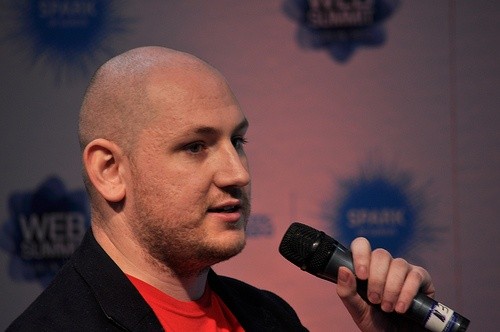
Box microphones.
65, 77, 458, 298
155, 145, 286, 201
278, 221, 470, 331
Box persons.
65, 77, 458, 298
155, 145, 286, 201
0, 45, 439, 331
